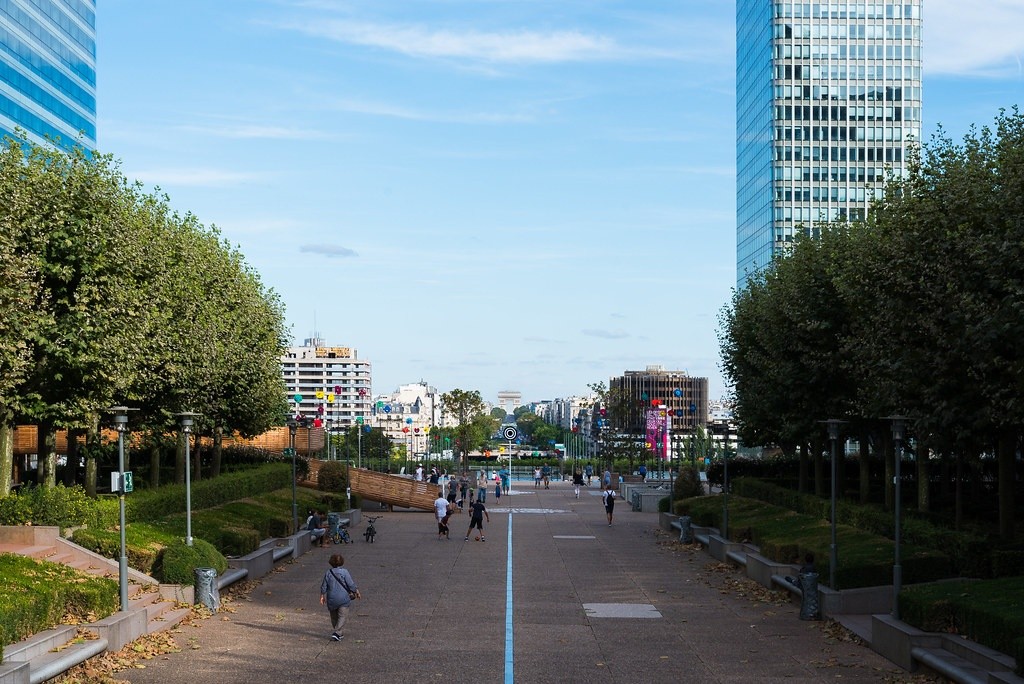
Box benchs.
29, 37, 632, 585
310, 534, 318, 542
339, 516, 350, 526
694, 534, 709, 546
771, 573, 803, 604
218, 567, 248, 589
28, 636, 109, 684
272, 546, 294, 565
726, 550, 748, 567
911, 646, 1011, 684
671, 521, 681, 530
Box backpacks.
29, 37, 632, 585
639, 466, 646, 473
605, 490, 615, 508
604, 471, 610, 483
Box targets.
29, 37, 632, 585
503, 426, 517, 440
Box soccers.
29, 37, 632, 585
475, 535, 480, 541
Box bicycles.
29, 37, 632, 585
362, 514, 383, 543
333, 524, 353, 544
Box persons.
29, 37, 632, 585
320, 554, 361, 641
468, 488, 474, 507
604, 468, 610, 489
477, 469, 488, 503
637, 464, 647, 481
434, 492, 451, 540
299, 414, 322, 428
586, 462, 592, 485
541, 463, 550, 489
306, 509, 330, 548
603, 485, 616, 527
464, 499, 489, 542
494, 481, 501, 505
413, 463, 448, 485
447, 475, 459, 511
573, 470, 584, 499
492, 471, 498, 480
534, 465, 541, 487
499, 464, 510, 496
459, 472, 472, 502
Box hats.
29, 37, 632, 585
469, 488, 474, 491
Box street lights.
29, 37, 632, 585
175, 410, 206, 546
340, 425, 355, 510
106, 407, 141, 612
716, 424, 737, 540
878, 414, 917, 623
818, 418, 852, 588
285, 417, 305, 535
669, 427, 678, 514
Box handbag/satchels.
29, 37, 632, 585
349, 590, 356, 600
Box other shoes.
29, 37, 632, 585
609, 525, 611, 526
482, 538, 485, 542
332, 632, 340, 641
464, 537, 468, 542
322, 543, 329, 547
341, 634, 343, 639
